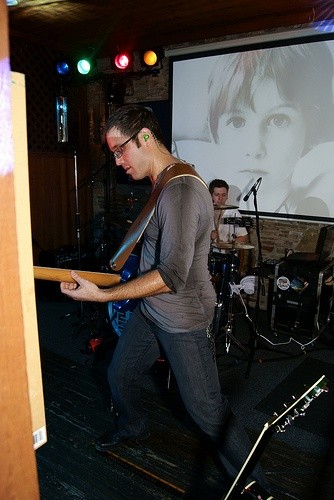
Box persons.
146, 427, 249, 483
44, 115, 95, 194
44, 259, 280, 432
59, 105, 285, 500
209, 180, 248, 245
208, 41, 334, 214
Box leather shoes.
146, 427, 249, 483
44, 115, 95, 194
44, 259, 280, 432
96, 424, 130, 453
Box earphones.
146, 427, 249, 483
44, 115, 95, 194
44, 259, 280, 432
144, 135, 148, 142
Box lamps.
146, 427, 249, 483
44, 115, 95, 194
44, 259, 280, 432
56, 47, 162, 76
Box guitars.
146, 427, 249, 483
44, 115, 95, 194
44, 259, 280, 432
33, 247, 139, 313
220, 373, 333, 500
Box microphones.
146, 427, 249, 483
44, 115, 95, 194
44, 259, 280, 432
243, 178, 262, 202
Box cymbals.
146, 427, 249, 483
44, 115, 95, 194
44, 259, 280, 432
210, 240, 255, 252
213, 200, 239, 211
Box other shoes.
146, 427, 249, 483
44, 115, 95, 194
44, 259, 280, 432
237, 318, 249, 345
216, 342, 230, 354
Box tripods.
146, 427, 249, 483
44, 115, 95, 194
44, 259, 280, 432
215, 190, 292, 380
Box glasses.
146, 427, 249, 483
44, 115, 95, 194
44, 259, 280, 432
114, 131, 139, 158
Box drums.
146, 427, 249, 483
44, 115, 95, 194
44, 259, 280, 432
208, 252, 241, 277
